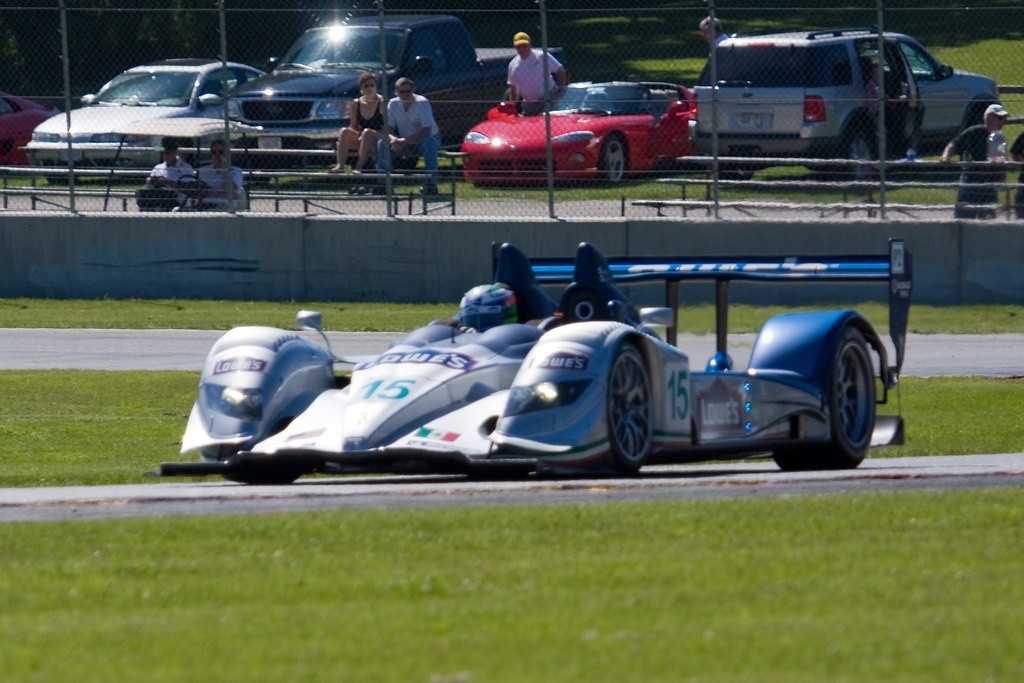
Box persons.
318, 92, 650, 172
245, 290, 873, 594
699, 16, 728, 44
375, 77, 441, 195
507, 32, 566, 116
459, 284, 517, 334
140, 136, 194, 211
329, 72, 383, 174
196, 139, 243, 212
940, 104, 1009, 220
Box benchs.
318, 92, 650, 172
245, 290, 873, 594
0, 147, 468, 216
631, 152, 1024, 219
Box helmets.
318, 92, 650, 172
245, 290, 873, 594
459, 283, 517, 333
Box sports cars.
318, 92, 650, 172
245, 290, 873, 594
461, 78, 697, 185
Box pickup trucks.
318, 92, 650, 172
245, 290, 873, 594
228, 12, 566, 187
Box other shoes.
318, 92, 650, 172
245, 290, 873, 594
327, 165, 345, 174
351, 169, 362, 174
371, 186, 393, 195
421, 183, 439, 199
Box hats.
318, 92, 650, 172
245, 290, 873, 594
983, 103, 1011, 120
513, 31, 530, 46
154, 135, 183, 152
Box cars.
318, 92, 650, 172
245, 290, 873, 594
24, 58, 271, 190
0, 94, 66, 167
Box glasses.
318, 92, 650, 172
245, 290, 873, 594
211, 149, 226, 156
516, 44, 528, 50
362, 83, 374, 87
399, 89, 411, 95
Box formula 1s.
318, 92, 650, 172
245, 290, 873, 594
150, 240, 922, 483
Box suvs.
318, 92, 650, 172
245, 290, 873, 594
686, 27, 1005, 190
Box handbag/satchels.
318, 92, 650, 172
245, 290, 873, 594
136, 176, 179, 209
178, 173, 214, 199
